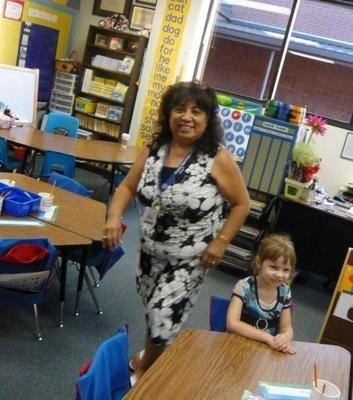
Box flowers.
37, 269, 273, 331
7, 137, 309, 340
288, 115, 328, 186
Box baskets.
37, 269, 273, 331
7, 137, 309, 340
0, 186, 42, 217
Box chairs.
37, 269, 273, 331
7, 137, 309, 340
73, 322, 131, 400
209, 296, 295, 332
0, 112, 104, 342
96, 165, 130, 202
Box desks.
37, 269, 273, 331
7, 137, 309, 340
308, 186, 352, 221
122, 328, 352, 400
0, 127, 143, 193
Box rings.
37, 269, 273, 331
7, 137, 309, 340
103, 236, 106, 240
211, 260, 216, 266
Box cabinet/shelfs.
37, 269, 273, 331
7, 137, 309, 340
218, 196, 283, 280
74, 24, 148, 142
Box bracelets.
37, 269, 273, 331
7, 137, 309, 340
218, 234, 229, 244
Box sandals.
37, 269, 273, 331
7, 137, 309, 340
129, 376, 138, 387
128, 349, 145, 371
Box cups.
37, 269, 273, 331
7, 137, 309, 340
121, 133, 130, 148
0, 178, 17, 188
37, 191, 55, 213
308, 379, 341, 400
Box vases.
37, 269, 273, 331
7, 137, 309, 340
285, 177, 314, 201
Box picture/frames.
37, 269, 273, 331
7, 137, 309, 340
92, 0, 133, 20
134, 0, 158, 7
129, 4, 155, 32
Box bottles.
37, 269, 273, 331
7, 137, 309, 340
301, 178, 318, 205
314, 189, 326, 205
265, 99, 306, 124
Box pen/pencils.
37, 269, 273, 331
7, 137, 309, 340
1, 191, 11, 200
314, 361, 317, 388
50, 180, 56, 197
8, 169, 16, 184
322, 384, 326, 393
0, 191, 5, 198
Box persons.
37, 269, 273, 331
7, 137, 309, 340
102, 80, 250, 383
226, 235, 296, 357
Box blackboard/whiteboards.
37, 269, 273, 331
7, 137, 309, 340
0, 63, 40, 127
